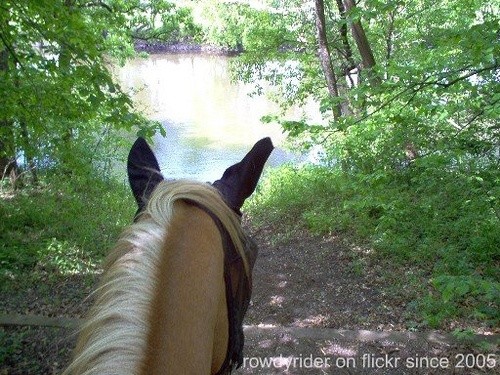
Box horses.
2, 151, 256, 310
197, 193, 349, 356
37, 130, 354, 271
56, 136, 276, 375
